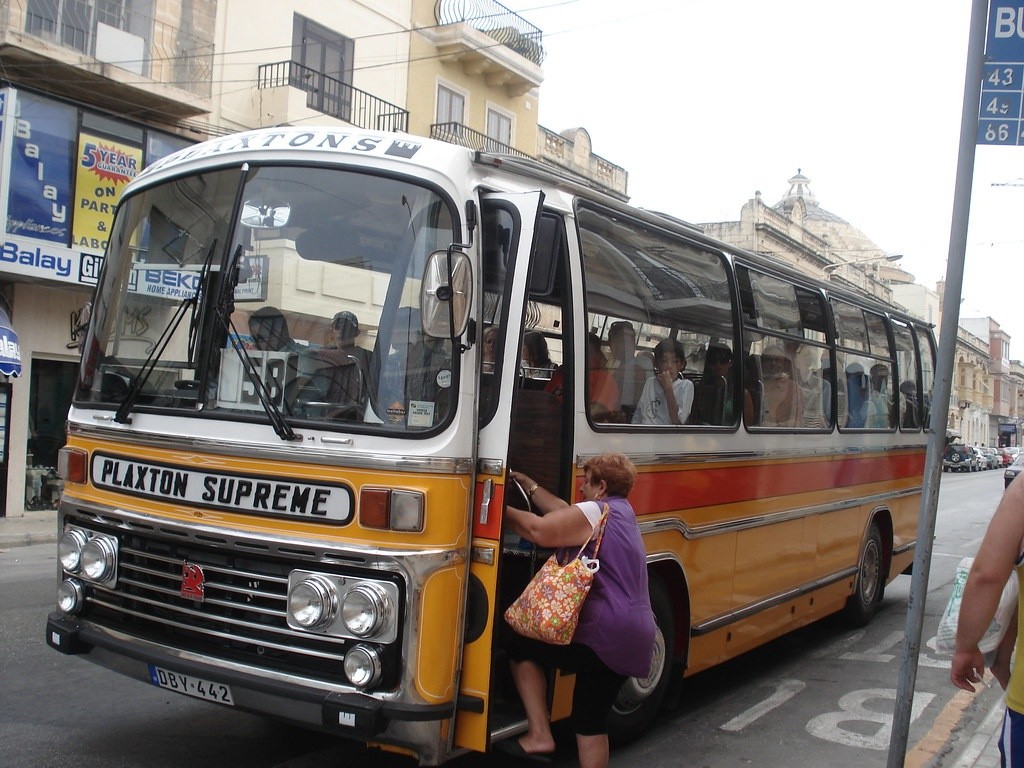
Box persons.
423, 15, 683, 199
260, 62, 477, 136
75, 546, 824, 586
493, 452, 656, 768
248, 306, 329, 416
321, 312, 373, 365
974, 442, 979, 447
950, 467, 1024, 768
483, 321, 931, 429
981, 443, 985, 447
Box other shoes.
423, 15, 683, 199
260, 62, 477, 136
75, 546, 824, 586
495, 740, 551, 761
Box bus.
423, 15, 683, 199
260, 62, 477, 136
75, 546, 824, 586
46, 126, 938, 768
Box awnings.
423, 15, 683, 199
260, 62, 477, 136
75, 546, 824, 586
0, 292, 23, 378
946, 429, 961, 443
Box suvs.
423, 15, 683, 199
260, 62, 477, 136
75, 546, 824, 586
942, 442, 979, 472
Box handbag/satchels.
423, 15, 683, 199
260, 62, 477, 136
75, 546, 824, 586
502, 503, 610, 645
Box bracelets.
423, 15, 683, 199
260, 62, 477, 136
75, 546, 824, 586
527, 483, 538, 499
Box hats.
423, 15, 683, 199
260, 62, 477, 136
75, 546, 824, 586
331, 311, 358, 328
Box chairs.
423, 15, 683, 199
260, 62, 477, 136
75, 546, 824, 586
481, 361, 556, 389
687, 355, 918, 429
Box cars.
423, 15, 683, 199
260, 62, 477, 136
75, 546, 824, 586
973, 448, 987, 471
981, 448, 999, 469
989, 448, 1004, 468
998, 448, 1013, 468
1004, 452, 1024, 489
1005, 447, 1020, 461
